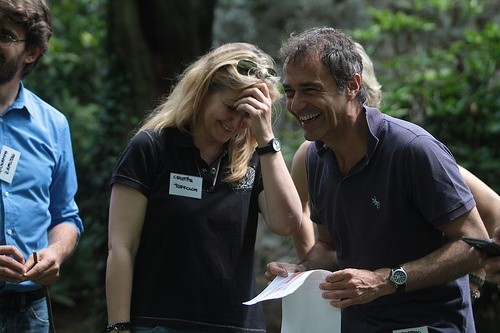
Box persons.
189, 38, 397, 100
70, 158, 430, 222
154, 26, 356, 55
263, 24, 488, 333
0, 0, 84, 333
286, 42, 500, 333
101, 43, 304, 333
469, 224, 500, 291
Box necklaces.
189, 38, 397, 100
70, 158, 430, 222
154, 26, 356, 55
193, 153, 221, 199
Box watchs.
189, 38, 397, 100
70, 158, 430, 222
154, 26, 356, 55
388, 263, 407, 294
255, 137, 282, 155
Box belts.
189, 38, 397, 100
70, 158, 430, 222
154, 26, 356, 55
1, 287, 46, 307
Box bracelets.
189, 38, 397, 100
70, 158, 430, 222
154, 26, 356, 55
106, 322, 132, 332
472, 290, 481, 299
469, 273, 485, 288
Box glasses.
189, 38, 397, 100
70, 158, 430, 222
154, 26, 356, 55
0, 33, 25, 47
233, 59, 278, 80
